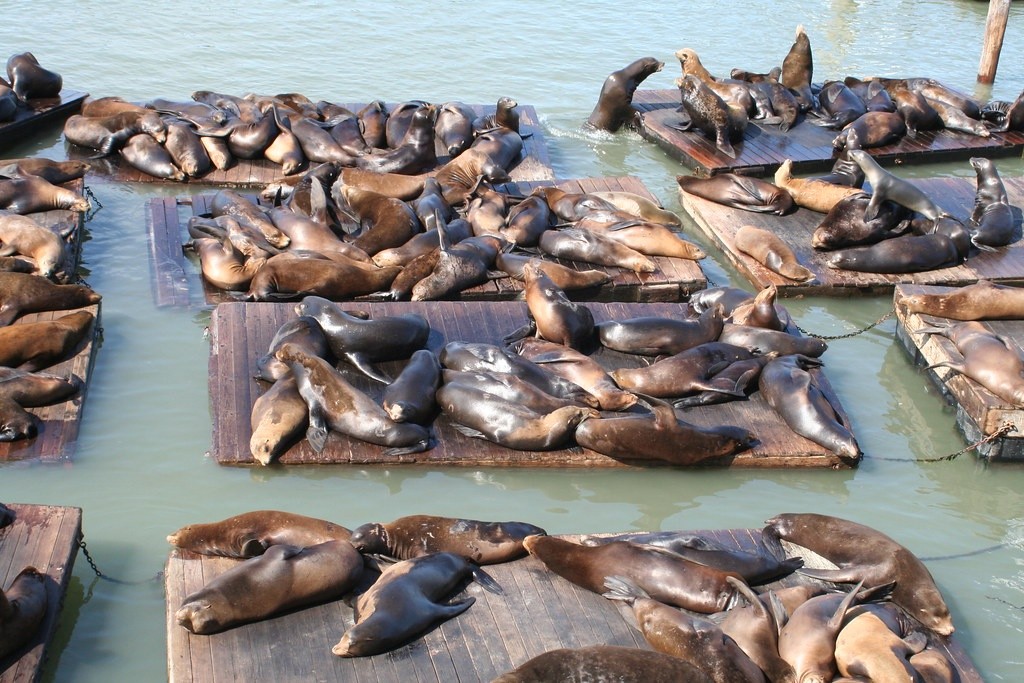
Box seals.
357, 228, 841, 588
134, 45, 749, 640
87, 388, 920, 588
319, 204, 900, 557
0, 31, 1023, 683
